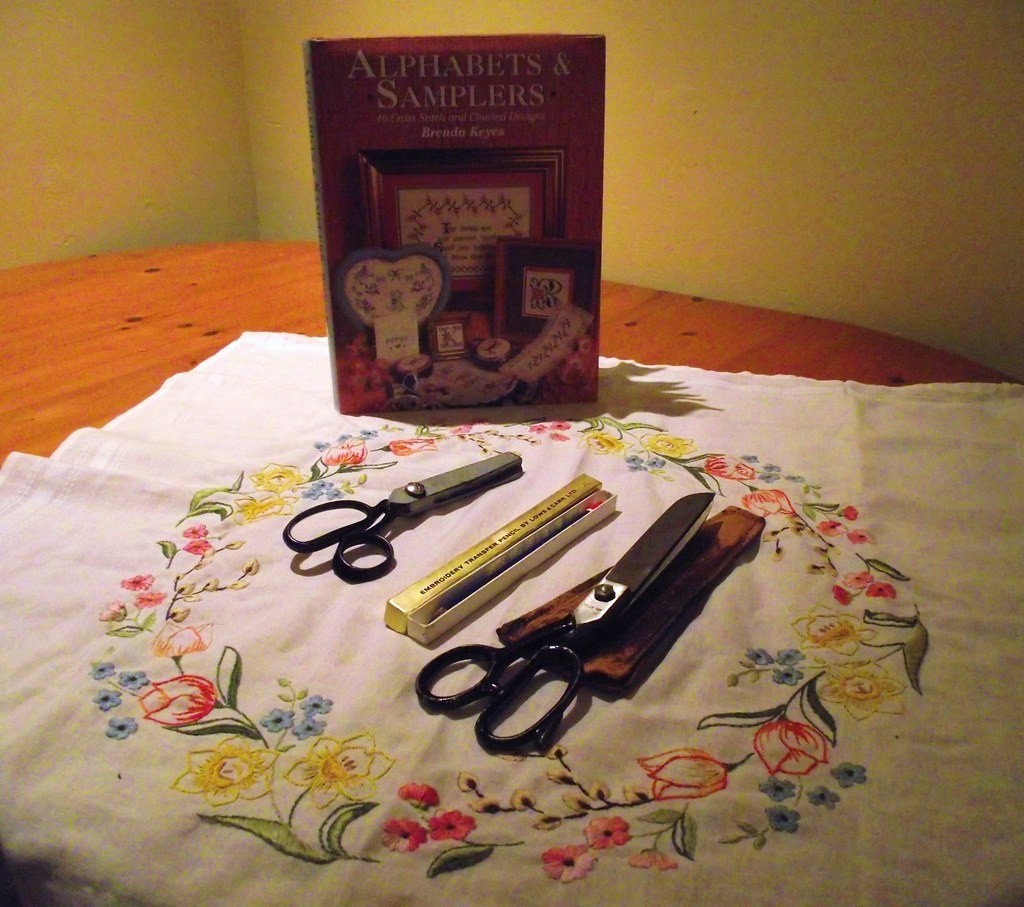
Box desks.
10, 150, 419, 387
0, 240, 1024, 907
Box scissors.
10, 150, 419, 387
281, 450, 523, 584
411, 490, 717, 756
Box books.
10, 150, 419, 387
304, 32, 606, 416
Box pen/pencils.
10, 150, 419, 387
425, 493, 608, 624
